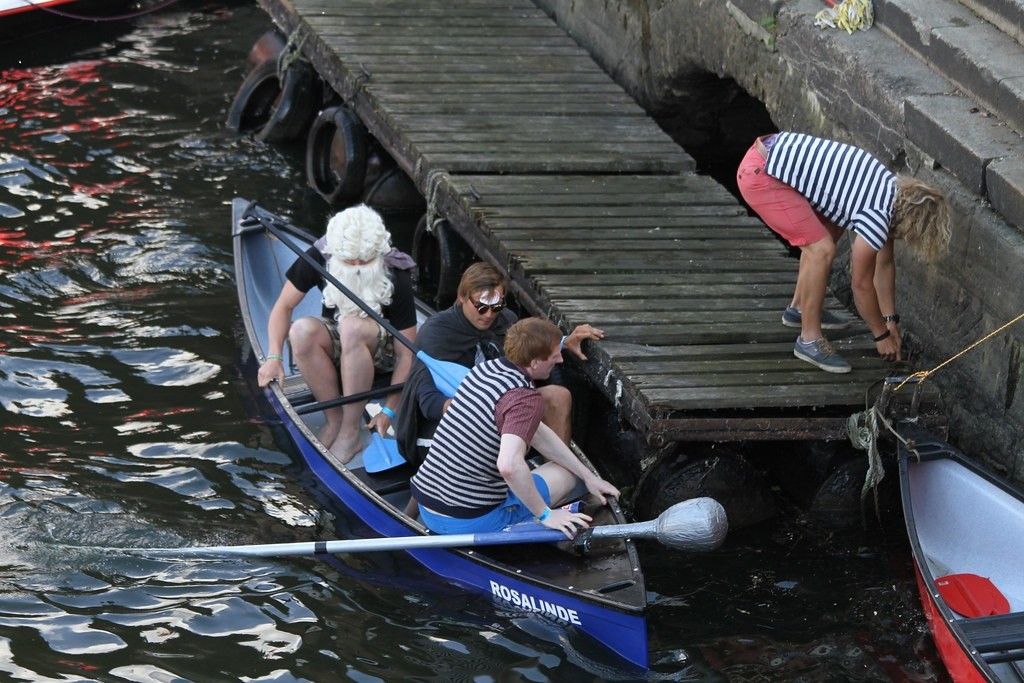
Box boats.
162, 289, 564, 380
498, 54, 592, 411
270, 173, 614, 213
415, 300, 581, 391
229, 197, 650, 671
879, 376, 1024, 683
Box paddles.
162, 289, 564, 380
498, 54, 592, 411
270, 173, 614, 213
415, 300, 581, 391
360, 407, 407, 474
238, 199, 471, 398
934, 573, 1024, 683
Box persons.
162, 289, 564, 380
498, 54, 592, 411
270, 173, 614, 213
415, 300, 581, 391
736, 130, 953, 374
396, 261, 605, 469
256, 203, 418, 465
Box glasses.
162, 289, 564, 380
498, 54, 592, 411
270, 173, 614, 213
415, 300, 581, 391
468, 295, 507, 314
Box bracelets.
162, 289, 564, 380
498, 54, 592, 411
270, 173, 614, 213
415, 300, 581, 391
267, 354, 283, 359
381, 406, 395, 418
872, 330, 890, 342
883, 314, 901, 323
407, 317, 621, 541
560, 335, 567, 349
537, 508, 552, 524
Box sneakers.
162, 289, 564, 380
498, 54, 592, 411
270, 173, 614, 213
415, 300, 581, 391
793, 336, 851, 373
782, 305, 851, 330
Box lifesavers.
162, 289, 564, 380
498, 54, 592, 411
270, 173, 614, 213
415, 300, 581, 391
225, 54, 319, 147
411, 210, 462, 307
307, 108, 366, 205
